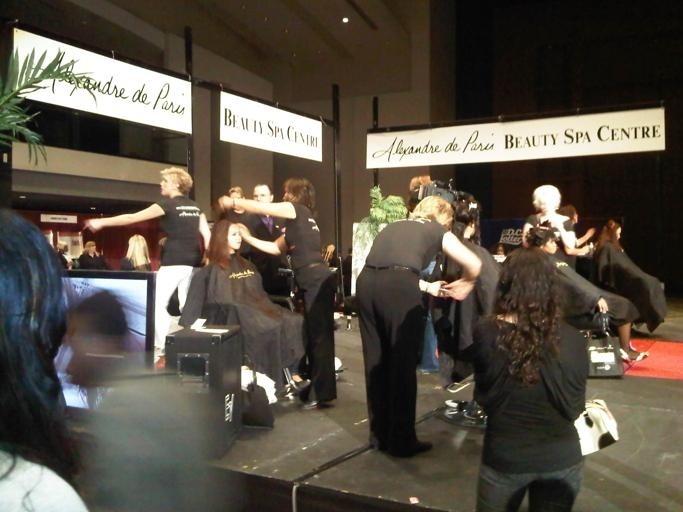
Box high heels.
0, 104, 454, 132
620, 344, 650, 367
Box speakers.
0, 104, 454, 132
165, 324, 242, 458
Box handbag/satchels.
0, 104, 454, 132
584, 336, 625, 378
576, 311, 623, 339
574, 398, 620, 457
239, 353, 277, 429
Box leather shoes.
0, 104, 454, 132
292, 377, 310, 391
302, 399, 321, 411
369, 438, 434, 458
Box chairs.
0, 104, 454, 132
152, 262, 312, 431
563, 308, 626, 379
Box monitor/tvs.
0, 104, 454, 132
52, 269, 156, 422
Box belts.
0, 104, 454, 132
293, 262, 321, 272
364, 264, 420, 277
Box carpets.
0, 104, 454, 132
617, 336, 682, 382
